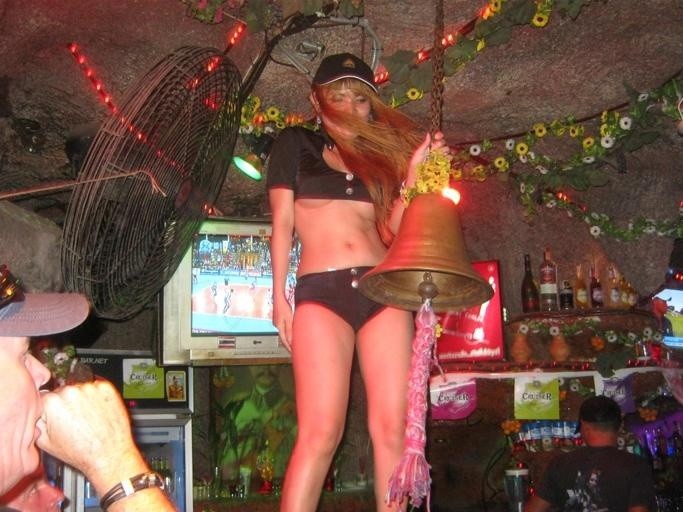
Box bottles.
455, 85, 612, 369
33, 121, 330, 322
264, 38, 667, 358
521, 254, 539, 313
574, 265, 589, 310
590, 264, 604, 308
147, 456, 174, 497
538, 247, 560, 312
559, 280, 574, 311
503, 468, 530, 512
608, 276, 640, 311
209, 466, 224, 499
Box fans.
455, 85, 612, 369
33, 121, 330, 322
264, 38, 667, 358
0, 45, 246, 321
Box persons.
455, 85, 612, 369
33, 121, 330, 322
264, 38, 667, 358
0, 265, 178, 512
268, 53, 453, 512
6, 473, 66, 512
523, 395, 654, 512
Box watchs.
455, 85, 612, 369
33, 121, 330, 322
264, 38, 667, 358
99, 470, 174, 512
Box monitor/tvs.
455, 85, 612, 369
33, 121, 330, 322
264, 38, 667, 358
156, 219, 304, 367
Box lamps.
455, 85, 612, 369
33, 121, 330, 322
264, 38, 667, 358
233, 132, 276, 182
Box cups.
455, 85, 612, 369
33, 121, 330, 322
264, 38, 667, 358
239, 465, 252, 497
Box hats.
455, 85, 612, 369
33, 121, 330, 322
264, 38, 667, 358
314, 52, 379, 94
0, 265, 90, 337
574, 395, 621, 434
